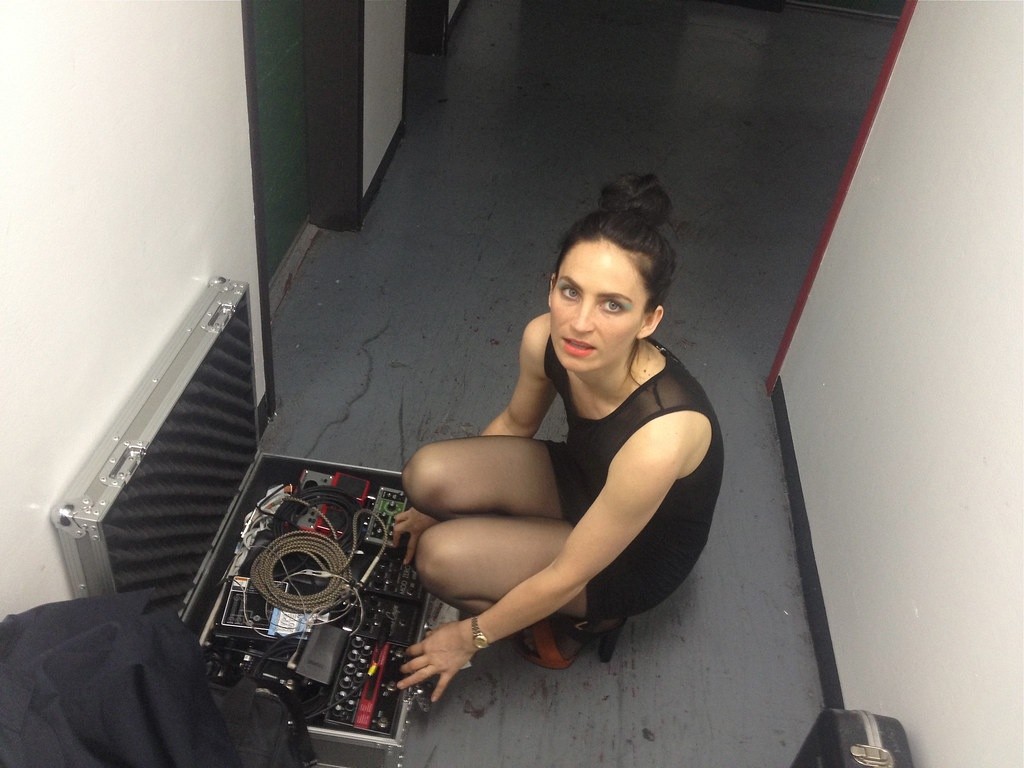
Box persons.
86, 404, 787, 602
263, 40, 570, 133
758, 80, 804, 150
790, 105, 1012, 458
396, 171, 726, 703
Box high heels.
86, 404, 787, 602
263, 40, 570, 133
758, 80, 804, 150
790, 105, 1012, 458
512, 616, 627, 670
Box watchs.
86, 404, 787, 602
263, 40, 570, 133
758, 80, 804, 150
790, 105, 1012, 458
472, 614, 492, 649
